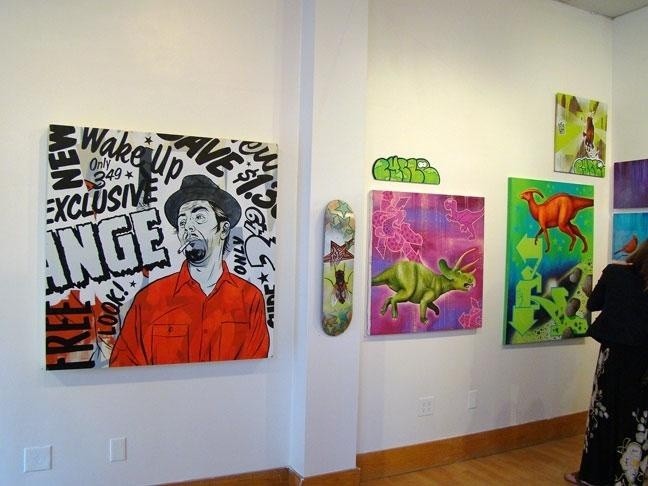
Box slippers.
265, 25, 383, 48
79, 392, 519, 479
564, 470, 588, 486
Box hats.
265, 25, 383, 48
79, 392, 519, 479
164, 175, 242, 231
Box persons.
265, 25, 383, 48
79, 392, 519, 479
565, 237, 648, 485
109, 173, 270, 369
